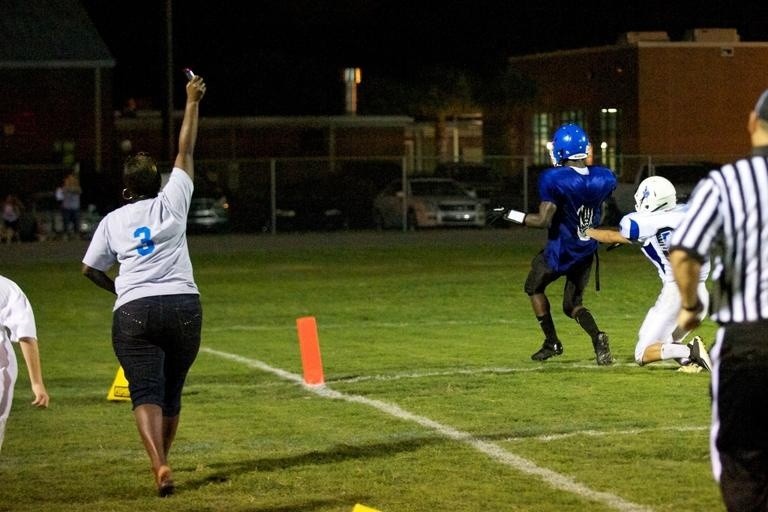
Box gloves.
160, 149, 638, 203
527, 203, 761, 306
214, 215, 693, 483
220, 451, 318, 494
579, 207, 594, 233
486, 207, 510, 224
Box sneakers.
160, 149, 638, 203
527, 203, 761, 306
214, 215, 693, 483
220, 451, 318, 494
532, 342, 563, 360
677, 336, 712, 373
592, 332, 612, 365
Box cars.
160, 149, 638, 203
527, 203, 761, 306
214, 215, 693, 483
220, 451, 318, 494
371, 178, 490, 233
510, 164, 557, 216
233, 177, 318, 234
186, 183, 232, 235
25, 191, 104, 241
302, 173, 377, 230
431, 162, 509, 207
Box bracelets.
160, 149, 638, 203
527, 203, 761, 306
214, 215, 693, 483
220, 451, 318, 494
682, 297, 701, 312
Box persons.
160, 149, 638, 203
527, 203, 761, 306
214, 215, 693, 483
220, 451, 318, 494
578, 176, 713, 373
0, 275, 50, 451
667, 90, 768, 512
56, 174, 82, 242
1, 193, 24, 246
82, 74, 207, 501
493, 124, 618, 366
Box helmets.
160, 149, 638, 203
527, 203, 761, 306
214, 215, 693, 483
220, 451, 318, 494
552, 124, 589, 164
634, 176, 677, 211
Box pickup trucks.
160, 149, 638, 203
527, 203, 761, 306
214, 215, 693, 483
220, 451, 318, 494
605, 160, 709, 227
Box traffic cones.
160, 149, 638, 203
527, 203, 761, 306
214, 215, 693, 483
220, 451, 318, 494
105, 364, 129, 402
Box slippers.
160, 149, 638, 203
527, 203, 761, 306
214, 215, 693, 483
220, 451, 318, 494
159, 480, 174, 497
